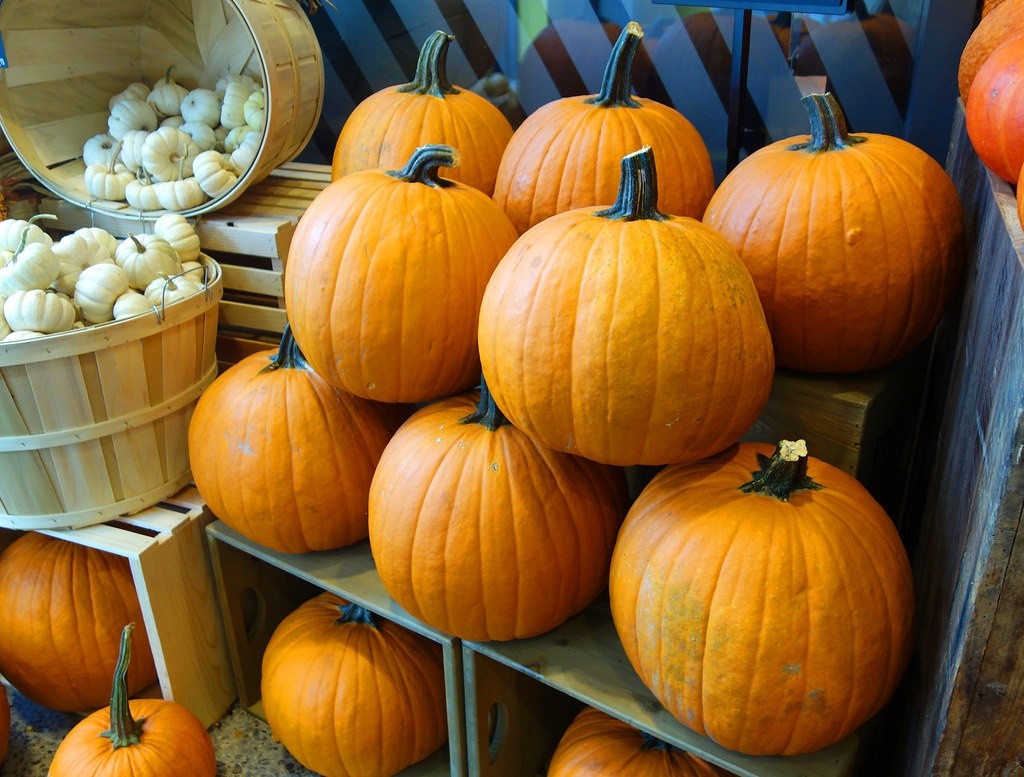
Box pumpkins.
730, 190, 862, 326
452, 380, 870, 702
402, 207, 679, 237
1, 0, 1024, 777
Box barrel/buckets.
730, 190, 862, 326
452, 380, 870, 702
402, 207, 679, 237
0, 251, 222, 530
1, 0, 324, 234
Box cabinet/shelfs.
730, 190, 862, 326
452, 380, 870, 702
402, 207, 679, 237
0, 92, 1024, 775
